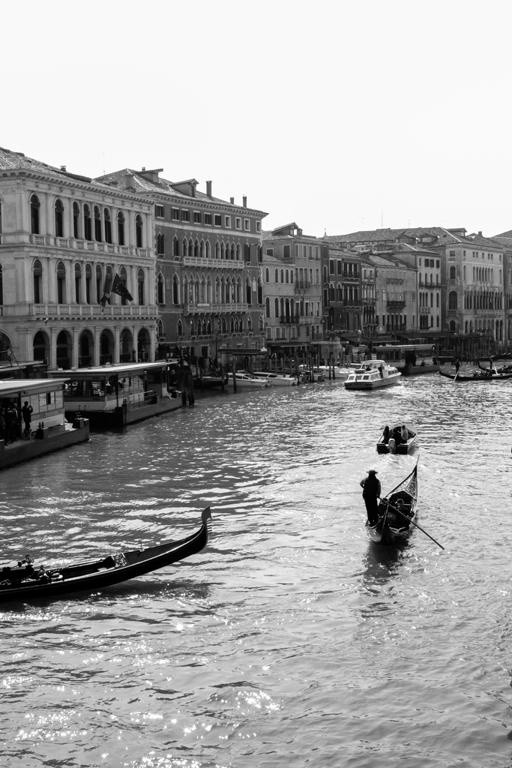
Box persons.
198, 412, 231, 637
382, 425, 409, 445
360, 471, 380, 528
455, 360, 460, 374
366, 365, 370, 370
420, 360, 425, 366
4, 403, 18, 446
22, 401, 33, 438
377, 364, 384, 378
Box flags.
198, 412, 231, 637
111, 273, 132, 301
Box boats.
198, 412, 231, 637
365, 454, 420, 545
0, 505, 213, 599
190, 363, 361, 387
344, 359, 402, 390
376, 425, 417, 453
438, 370, 512, 381
477, 362, 512, 372
